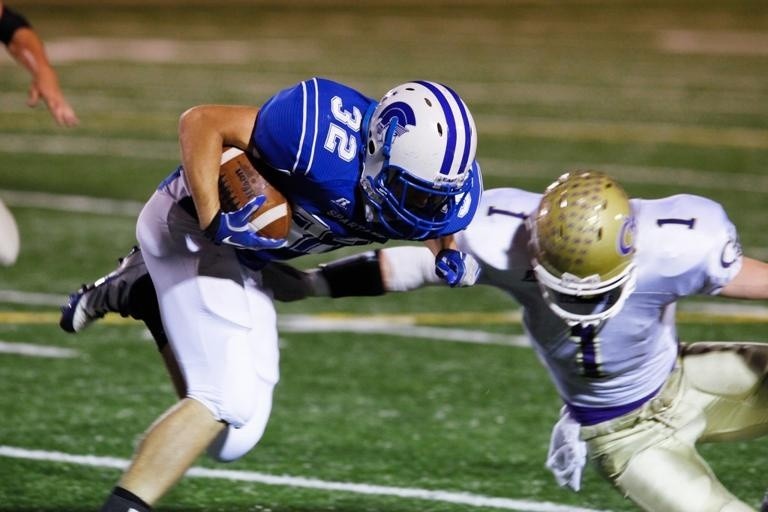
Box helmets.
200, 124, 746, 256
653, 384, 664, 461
356, 77, 485, 218
525, 167, 641, 301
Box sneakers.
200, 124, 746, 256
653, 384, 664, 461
59, 245, 149, 333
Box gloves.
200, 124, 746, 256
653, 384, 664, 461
261, 261, 307, 302
434, 247, 483, 289
200, 194, 289, 252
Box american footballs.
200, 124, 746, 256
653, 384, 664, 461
218, 145, 292, 239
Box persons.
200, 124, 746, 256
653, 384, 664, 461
236, 169, 768, 512
0, 0, 80, 268
59, 77, 483, 512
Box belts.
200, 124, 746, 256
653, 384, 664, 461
577, 352, 685, 443
